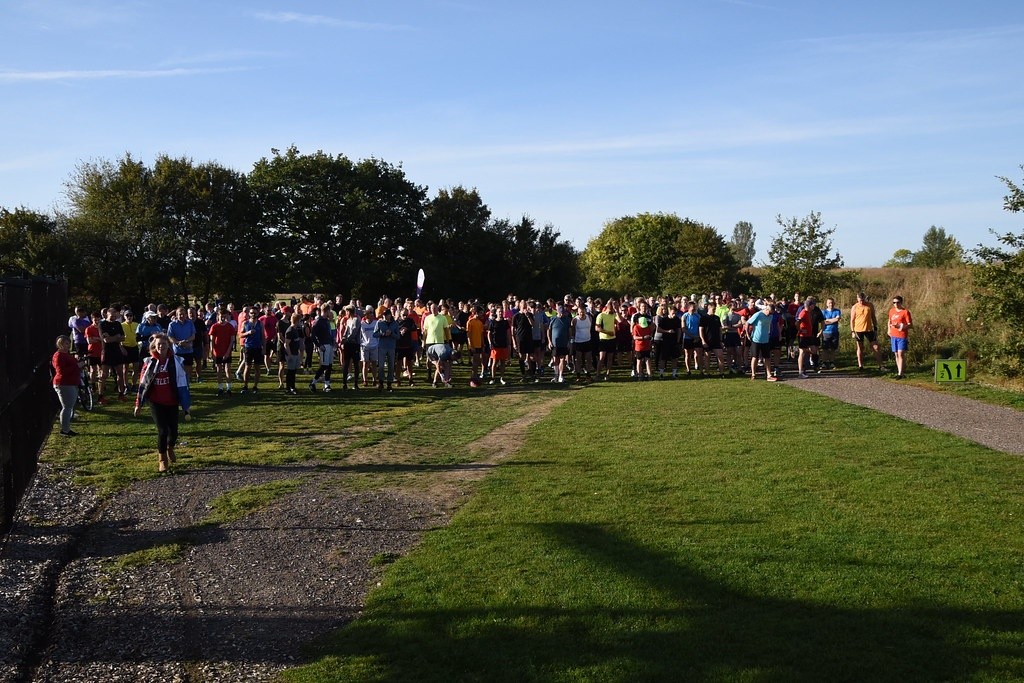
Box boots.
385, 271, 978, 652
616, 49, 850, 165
159, 451, 168, 472
167, 445, 176, 461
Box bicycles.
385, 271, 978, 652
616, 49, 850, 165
69, 351, 94, 412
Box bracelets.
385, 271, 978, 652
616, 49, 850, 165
384, 330, 387, 335
642, 336, 644, 340
898, 323, 903, 331
851, 330, 854, 333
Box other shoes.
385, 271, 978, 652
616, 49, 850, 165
378, 384, 382, 390
218, 389, 223, 396
895, 374, 902, 380
228, 390, 232, 396
60, 430, 78, 437
279, 384, 283, 389
373, 381, 377, 386
387, 386, 392, 390
363, 381, 367, 387
118, 396, 128, 401
98, 398, 109, 405
292, 388, 298, 394
396, 365, 837, 388
343, 385, 348, 391
235, 371, 242, 380
241, 386, 247, 394
858, 366, 865, 373
197, 377, 203, 382
252, 387, 257, 393
309, 383, 316, 393
323, 386, 332, 392
355, 385, 359, 390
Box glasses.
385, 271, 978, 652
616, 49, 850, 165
126, 315, 132, 317
893, 302, 901, 304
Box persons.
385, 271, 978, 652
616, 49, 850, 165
133, 334, 191, 473
53, 290, 914, 435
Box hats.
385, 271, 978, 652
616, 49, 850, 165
145, 311, 158, 318
639, 317, 648, 328
382, 310, 392, 315
124, 310, 133, 316
